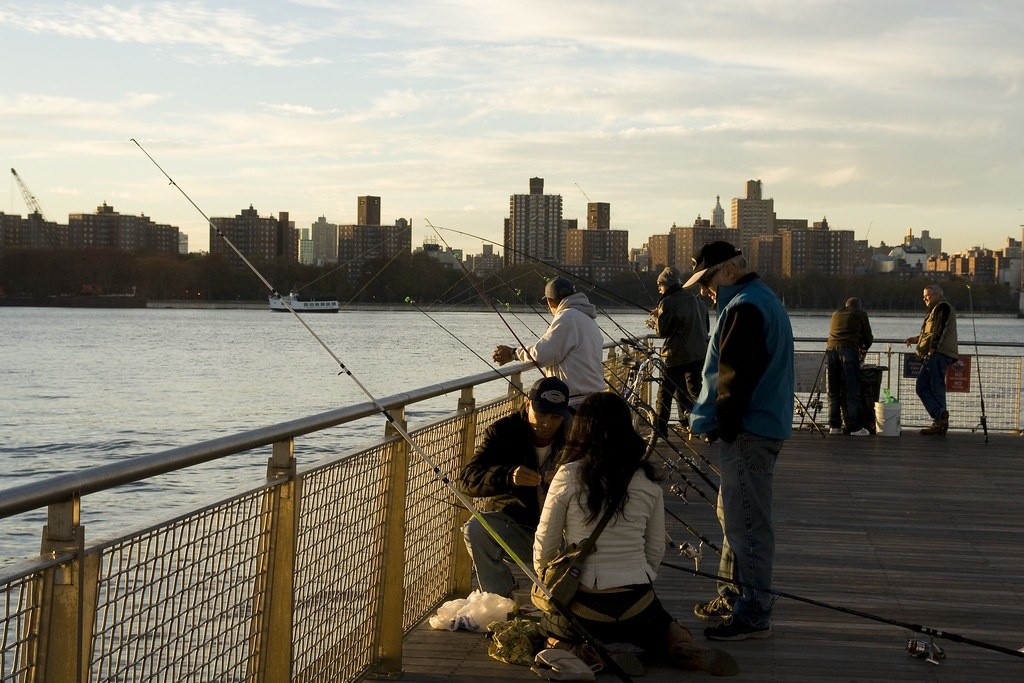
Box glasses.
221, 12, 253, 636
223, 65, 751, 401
700, 264, 725, 288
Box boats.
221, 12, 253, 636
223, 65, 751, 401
268, 292, 339, 313
0, 282, 148, 309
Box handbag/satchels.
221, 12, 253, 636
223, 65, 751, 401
531, 538, 598, 615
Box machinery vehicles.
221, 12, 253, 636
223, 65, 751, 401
10, 168, 82, 296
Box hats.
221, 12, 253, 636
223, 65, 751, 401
541, 276, 573, 299
530, 376, 571, 420
681, 240, 742, 288
657, 267, 680, 286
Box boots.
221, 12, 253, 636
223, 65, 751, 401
553, 641, 647, 676
666, 624, 740, 676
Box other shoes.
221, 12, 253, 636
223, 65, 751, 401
673, 425, 690, 434
920, 420, 942, 434
642, 434, 666, 443
934, 411, 950, 435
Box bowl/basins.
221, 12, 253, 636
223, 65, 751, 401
511, 589, 531, 605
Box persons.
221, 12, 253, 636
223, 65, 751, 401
531, 392, 740, 676
903, 285, 959, 434
825, 297, 873, 436
457, 376, 579, 597
643, 266, 711, 442
680, 240, 796, 640
491, 276, 603, 412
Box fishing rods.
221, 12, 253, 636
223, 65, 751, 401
402, 295, 723, 572
421, 177, 722, 511
791, 218, 875, 438
966, 285, 990, 443
417, 488, 1023, 669
126, 135, 631, 682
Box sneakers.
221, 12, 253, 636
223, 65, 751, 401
829, 428, 843, 435
704, 615, 772, 640
850, 427, 870, 436
694, 595, 735, 620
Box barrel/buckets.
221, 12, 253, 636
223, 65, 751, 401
873, 401, 902, 437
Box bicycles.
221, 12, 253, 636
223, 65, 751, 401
618, 337, 667, 463
